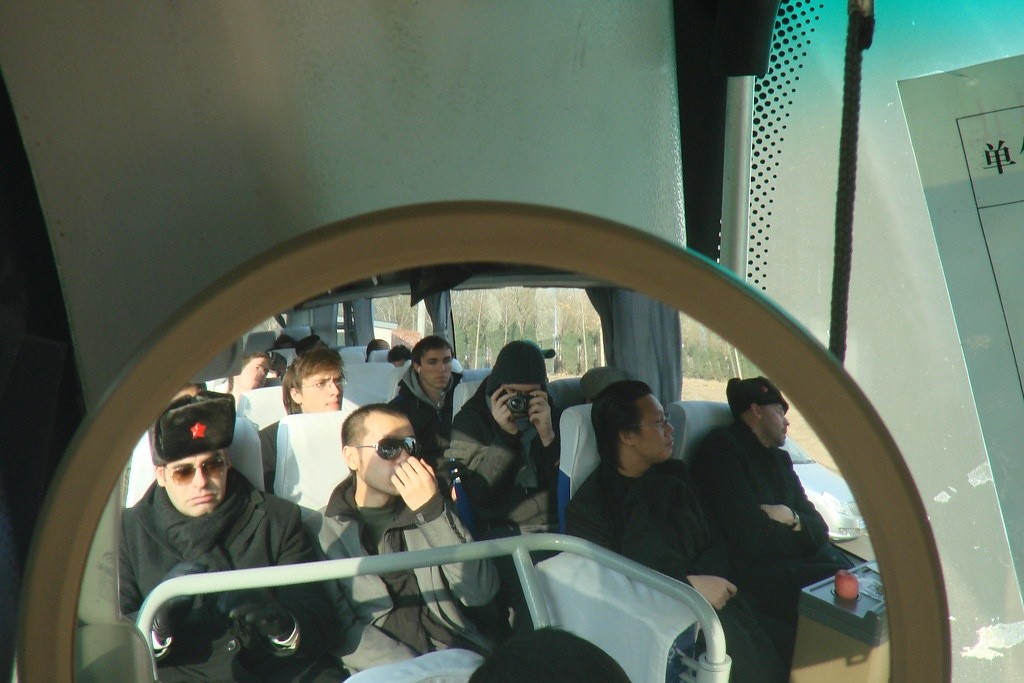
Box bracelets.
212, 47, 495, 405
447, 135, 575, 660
788, 510, 799, 529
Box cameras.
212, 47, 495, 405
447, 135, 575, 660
506, 391, 536, 417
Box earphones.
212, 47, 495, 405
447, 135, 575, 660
417, 367, 421, 375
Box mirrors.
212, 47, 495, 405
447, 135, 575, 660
12, 190, 964, 683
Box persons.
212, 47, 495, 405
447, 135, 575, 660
222, 335, 628, 642
118, 381, 632, 683
565, 375, 830, 683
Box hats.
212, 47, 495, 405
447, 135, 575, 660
148, 390, 236, 466
487, 341, 548, 385
725, 376, 788, 416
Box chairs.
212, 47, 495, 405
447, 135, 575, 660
280, 325, 312, 343
338, 348, 365, 362
338, 361, 399, 406
271, 347, 296, 367
239, 387, 357, 427
245, 332, 276, 356
666, 397, 738, 463
367, 349, 389, 362
548, 376, 589, 405
387, 358, 462, 411
403, 358, 464, 373
460, 368, 503, 384
451, 379, 484, 426
550, 402, 598, 536
124, 416, 266, 508
270, 412, 353, 513
341, 343, 367, 360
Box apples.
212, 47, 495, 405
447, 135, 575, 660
833, 568, 859, 598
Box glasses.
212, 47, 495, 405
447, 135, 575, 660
355, 435, 418, 460
300, 372, 349, 391
632, 413, 671, 429
165, 454, 227, 486
